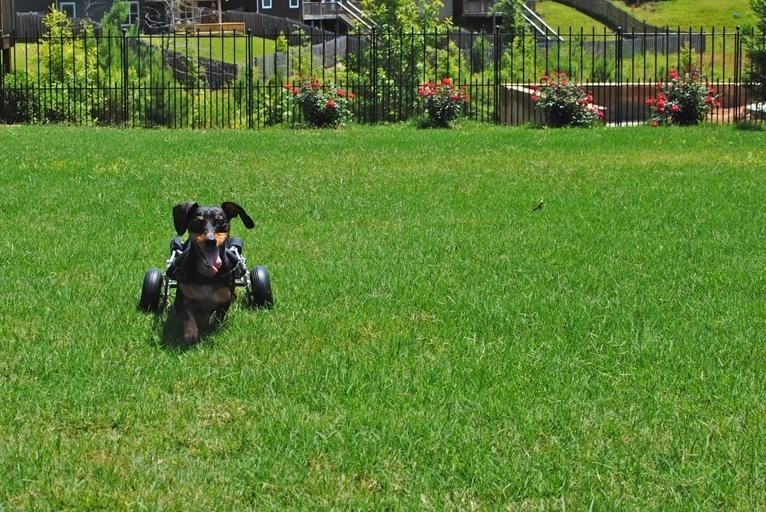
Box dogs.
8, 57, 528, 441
164, 201, 254, 347
